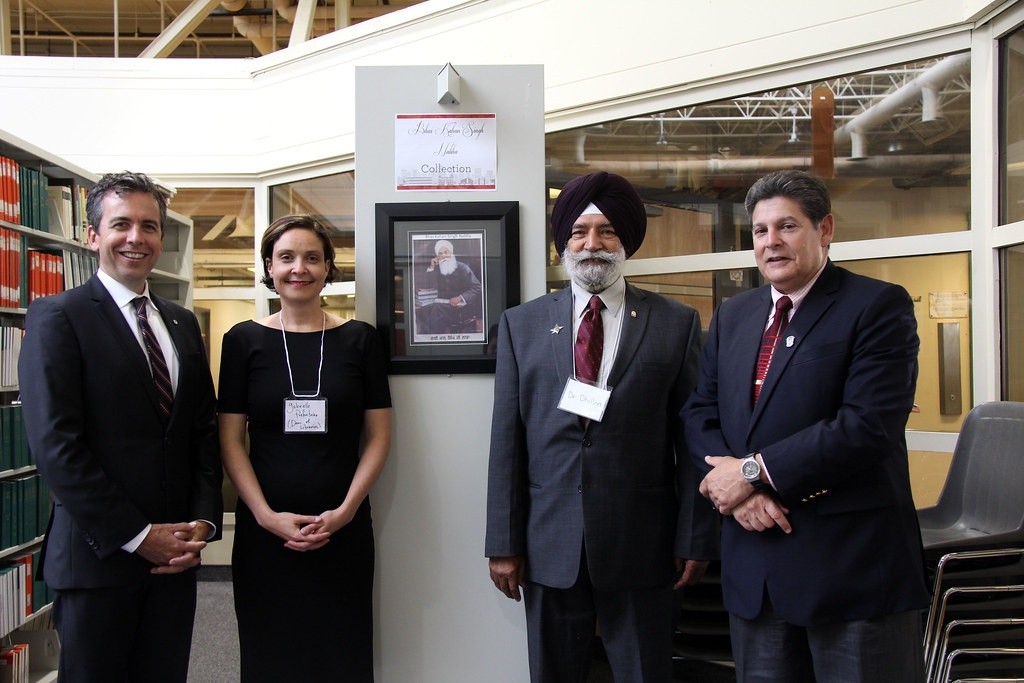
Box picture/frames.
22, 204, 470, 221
375, 201, 520, 374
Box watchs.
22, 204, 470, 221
741, 452, 773, 494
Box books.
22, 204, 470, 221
0, 157, 177, 683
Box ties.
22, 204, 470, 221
751, 296, 794, 411
574, 296, 608, 387
130, 295, 174, 414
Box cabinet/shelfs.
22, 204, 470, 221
0, 130, 191, 683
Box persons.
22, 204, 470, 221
424, 240, 483, 334
485, 172, 703, 683
218, 214, 391, 683
19, 172, 223, 683
683, 169, 932, 683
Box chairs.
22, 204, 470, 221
668, 398, 1024, 683
